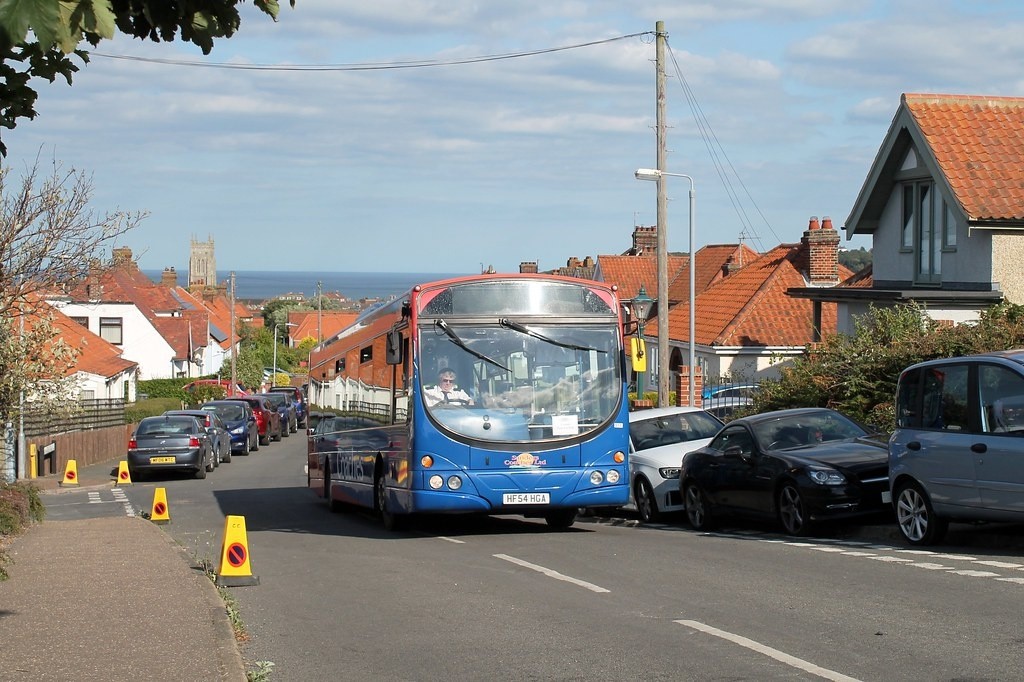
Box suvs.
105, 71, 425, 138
268, 386, 307, 424
160, 410, 232, 467
224, 395, 282, 446
180, 379, 250, 397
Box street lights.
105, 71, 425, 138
272, 323, 298, 387
635, 167, 696, 407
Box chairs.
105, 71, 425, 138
990, 394, 1024, 432
663, 419, 695, 442
147, 426, 165, 435
184, 427, 192, 434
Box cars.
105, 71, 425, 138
701, 383, 760, 409
679, 407, 893, 537
200, 402, 259, 456
254, 393, 298, 437
577, 406, 730, 523
313, 417, 400, 474
128, 415, 215, 481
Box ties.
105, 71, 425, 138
441, 391, 449, 404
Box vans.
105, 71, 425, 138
889, 348, 1024, 547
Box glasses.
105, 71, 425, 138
443, 379, 453, 383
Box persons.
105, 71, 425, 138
404, 368, 474, 406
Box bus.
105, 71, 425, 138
306, 274, 646, 528
